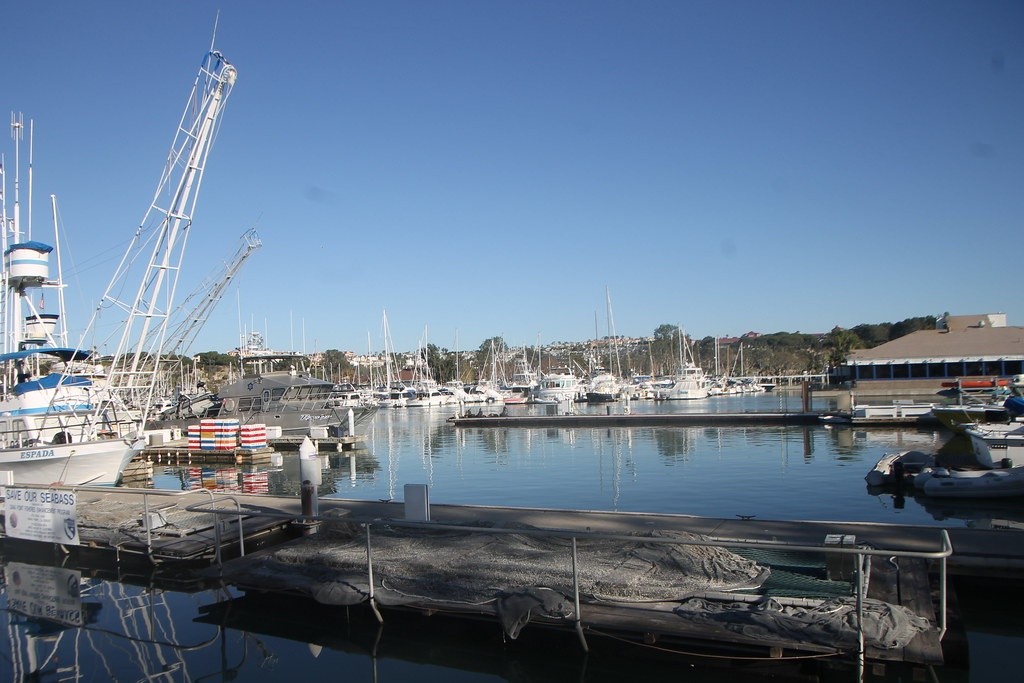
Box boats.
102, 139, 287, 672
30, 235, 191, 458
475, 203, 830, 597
967, 417, 1024, 469
0, 9, 237, 486
930, 375, 1024, 434
138, 311, 379, 438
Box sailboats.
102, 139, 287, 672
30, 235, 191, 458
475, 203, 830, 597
535, 283, 770, 402
332, 309, 534, 408
